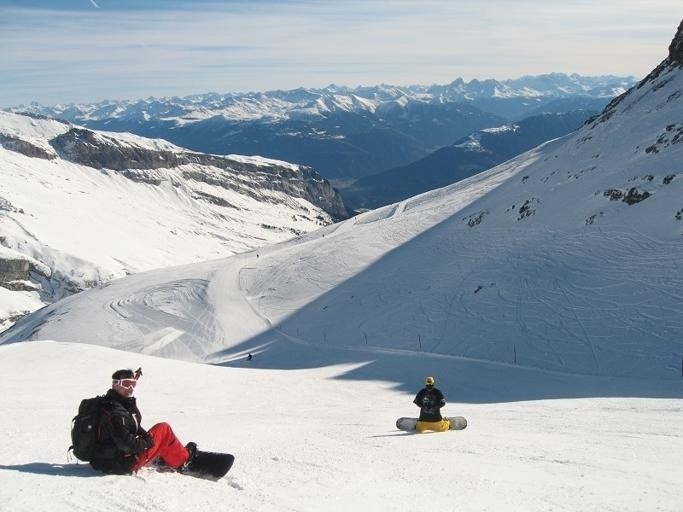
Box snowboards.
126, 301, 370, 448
396, 417, 467, 431
152, 450, 234, 481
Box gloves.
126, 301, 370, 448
143, 435, 152, 448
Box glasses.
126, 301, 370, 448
112, 378, 138, 390
426, 385, 434, 387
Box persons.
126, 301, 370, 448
87, 366, 196, 475
245, 353, 252, 361
412, 374, 451, 434
133, 366, 143, 381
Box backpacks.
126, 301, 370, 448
72, 395, 124, 462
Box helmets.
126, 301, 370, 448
426, 377, 434, 385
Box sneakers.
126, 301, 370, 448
175, 442, 196, 474
